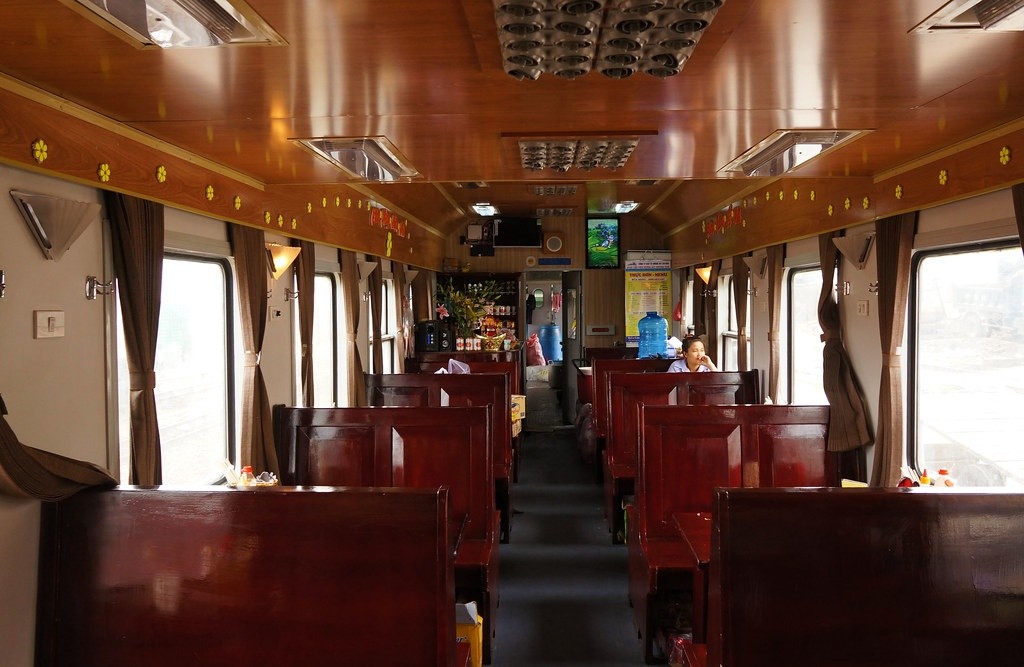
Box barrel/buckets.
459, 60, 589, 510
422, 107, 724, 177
637, 309, 668, 359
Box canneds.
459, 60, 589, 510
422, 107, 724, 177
456, 281, 518, 350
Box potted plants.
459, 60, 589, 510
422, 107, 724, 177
432, 276, 502, 350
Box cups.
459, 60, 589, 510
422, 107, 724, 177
504, 339, 511, 350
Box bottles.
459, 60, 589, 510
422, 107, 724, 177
934, 468, 952, 487
237, 466, 257, 487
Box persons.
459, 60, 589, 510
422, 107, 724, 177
666, 334, 720, 373
526, 287, 537, 340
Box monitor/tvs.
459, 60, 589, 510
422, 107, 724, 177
585, 214, 621, 270
493, 217, 542, 249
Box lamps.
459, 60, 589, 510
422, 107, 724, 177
608, 200, 640, 214
10, 188, 101, 262
264, 242, 302, 297
357, 261, 379, 283
468, 202, 501, 217
694, 265, 712, 285
832, 230, 876, 271
404, 270, 419, 285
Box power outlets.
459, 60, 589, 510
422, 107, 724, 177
33, 310, 66, 339
857, 300, 869, 316
742, 254, 768, 280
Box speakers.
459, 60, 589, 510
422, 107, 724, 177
543, 232, 566, 257
437, 320, 456, 352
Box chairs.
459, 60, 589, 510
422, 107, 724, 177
34, 345, 1023, 667
